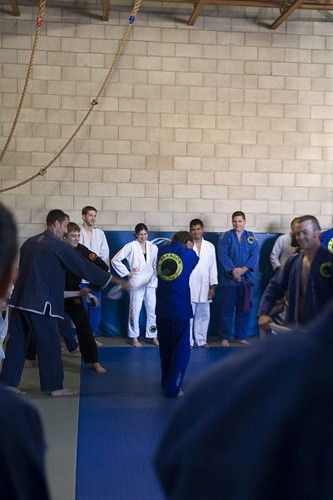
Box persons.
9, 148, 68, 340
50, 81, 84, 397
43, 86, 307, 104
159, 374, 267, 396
0, 206, 333, 500
